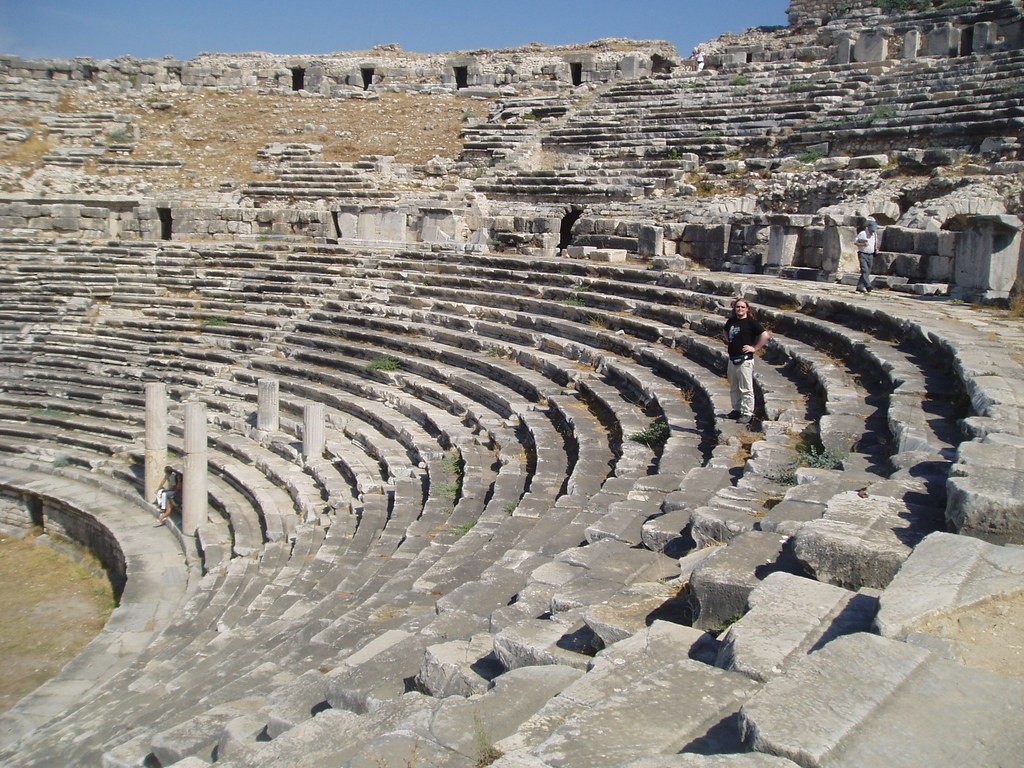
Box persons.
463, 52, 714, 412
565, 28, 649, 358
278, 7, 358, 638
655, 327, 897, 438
854, 223, 877, 292
695, 47, 705, 71
156, 465, 183, 521
722, 298, 769, 423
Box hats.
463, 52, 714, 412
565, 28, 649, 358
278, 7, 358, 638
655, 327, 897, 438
869, 222, 877, 232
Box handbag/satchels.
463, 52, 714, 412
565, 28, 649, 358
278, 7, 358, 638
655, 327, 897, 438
729, 353, 752, 365
874, 249, 878, 257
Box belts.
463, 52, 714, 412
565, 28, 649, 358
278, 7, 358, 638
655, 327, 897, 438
859, 251, 873, 255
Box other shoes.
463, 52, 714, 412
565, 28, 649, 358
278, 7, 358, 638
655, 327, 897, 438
867, 286, 873, 292
737, 414, 752, 423
857, 288, 867, 294
723, 410, 742, 418
